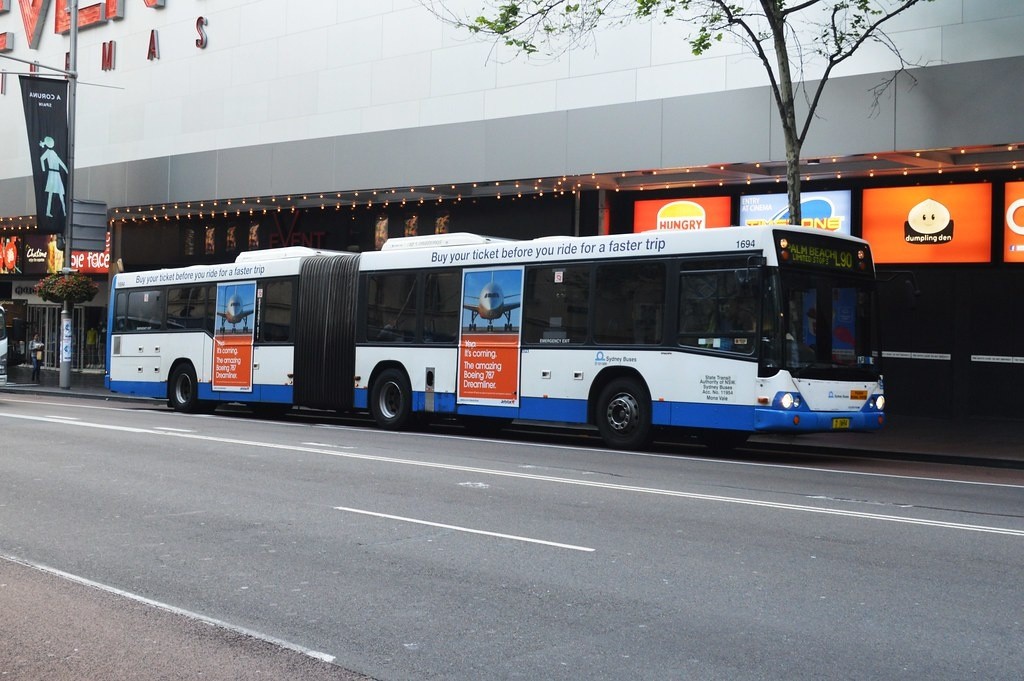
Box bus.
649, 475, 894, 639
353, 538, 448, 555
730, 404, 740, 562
104, 224, 888, 451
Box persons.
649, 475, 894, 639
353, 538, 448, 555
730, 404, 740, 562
29, 333, 44, 383
86, 319, 106, 368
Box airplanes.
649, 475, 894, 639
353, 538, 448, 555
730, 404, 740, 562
464, 272, 521, 332
217, 286, 254, 333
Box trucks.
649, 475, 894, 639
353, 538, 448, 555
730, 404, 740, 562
0, 306, 9, 387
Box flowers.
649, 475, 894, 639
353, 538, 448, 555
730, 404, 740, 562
33, 273, 101, 304
